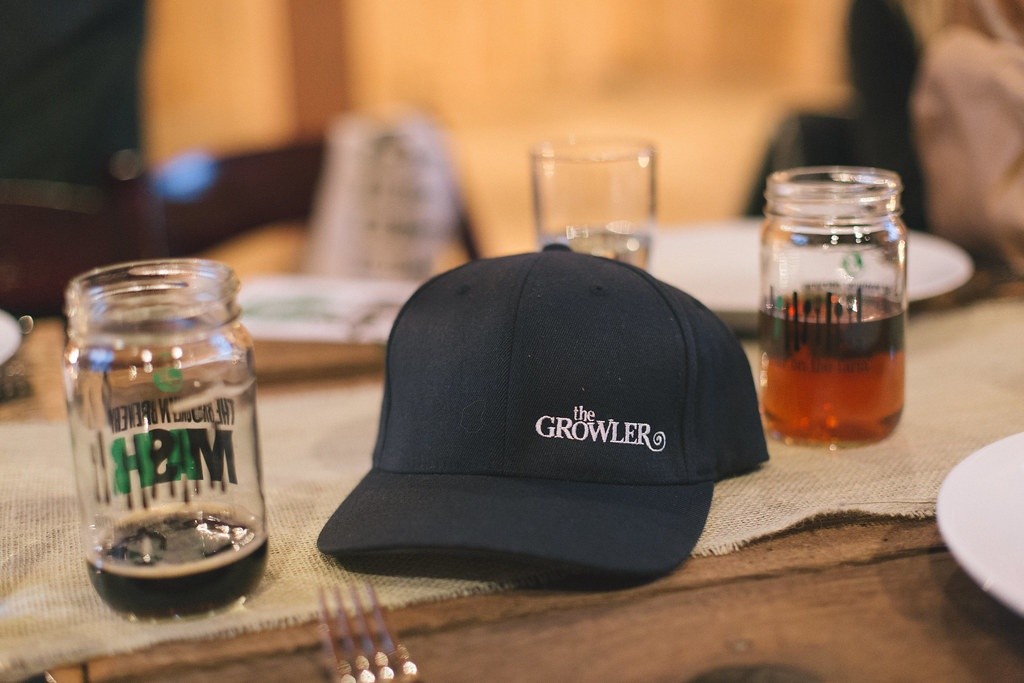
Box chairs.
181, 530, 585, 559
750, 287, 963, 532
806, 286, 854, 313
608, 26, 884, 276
0, 134, 479, 317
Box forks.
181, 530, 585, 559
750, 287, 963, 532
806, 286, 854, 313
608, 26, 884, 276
315, 578, 422, 683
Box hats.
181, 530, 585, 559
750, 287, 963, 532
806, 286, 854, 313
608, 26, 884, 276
316, 243, 770, 572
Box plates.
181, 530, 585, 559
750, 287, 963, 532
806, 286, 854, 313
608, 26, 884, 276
0, 308, 22, 365
937, 432, 1024, 618
655, 221, 976, 339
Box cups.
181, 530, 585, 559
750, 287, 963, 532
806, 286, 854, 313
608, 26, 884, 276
529, 134, 658, 273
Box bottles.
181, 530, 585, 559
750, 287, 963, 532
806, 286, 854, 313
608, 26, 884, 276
758, 164, 910, 450
60, 259, 271, 619
